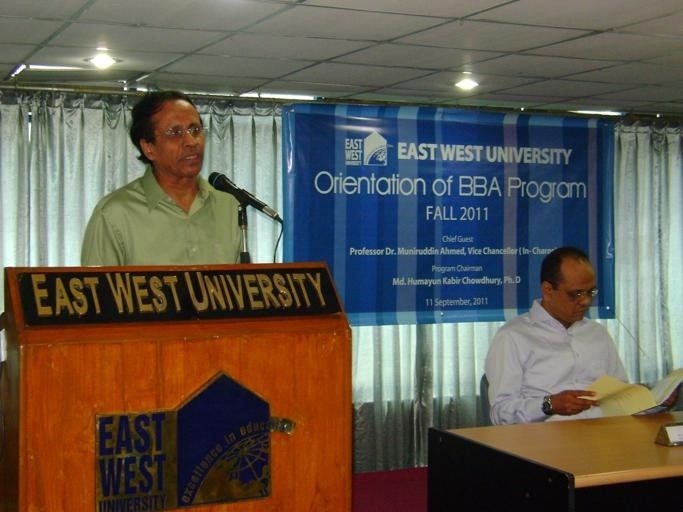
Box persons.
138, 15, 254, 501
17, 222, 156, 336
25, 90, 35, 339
81, 90, 248, 267
484, 246, 679, 426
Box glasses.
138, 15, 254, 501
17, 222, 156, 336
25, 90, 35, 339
556, 285, 598, 300
158, 127, 207, 139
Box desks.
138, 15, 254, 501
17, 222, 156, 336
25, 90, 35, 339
427, 411, 683, 512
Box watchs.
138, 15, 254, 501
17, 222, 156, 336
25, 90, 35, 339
541, 395, 555, 417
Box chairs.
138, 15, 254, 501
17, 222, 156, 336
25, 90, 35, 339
480, 374, 505, 424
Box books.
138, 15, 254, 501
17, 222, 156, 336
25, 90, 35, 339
575, 367, 682, 417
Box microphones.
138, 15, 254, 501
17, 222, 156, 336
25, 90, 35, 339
208, 171, 281, 222
